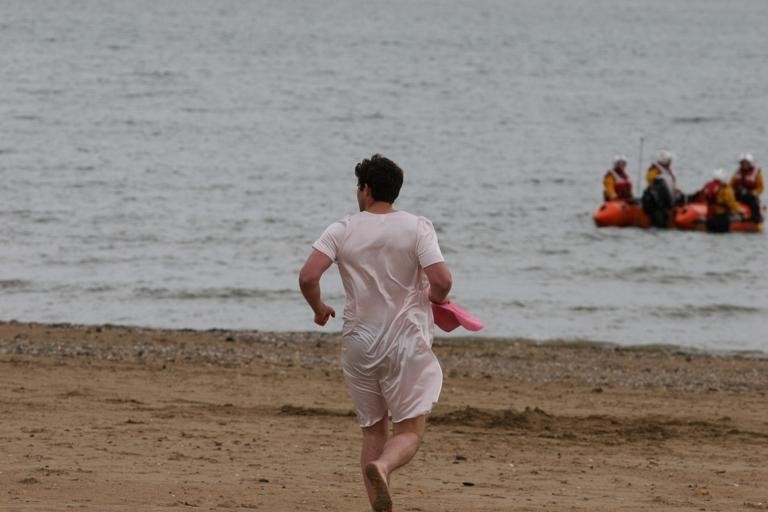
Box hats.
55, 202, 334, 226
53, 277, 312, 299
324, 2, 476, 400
432, 302, 484, 333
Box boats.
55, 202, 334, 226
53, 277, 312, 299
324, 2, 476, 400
593, 197, 765, 232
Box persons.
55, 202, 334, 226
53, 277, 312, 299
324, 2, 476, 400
641, 174, 675, 231
728, 152, 766, 232
601, 154, 639, 206
701, 167, 746, 235
646, 150, 686, 207
298, 152, 454, 512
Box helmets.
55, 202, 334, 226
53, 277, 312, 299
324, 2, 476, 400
614, 156, 627, 169
714, 169, 727, 185
740, 154, 753, 167
657, 151, 672, 164
